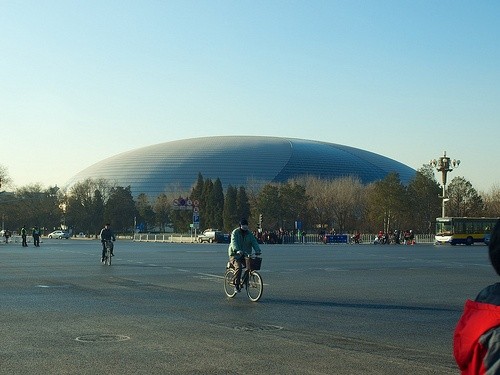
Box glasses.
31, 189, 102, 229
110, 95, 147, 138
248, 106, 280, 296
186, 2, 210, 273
106, 224, 110, 225
241, 222, 248, 226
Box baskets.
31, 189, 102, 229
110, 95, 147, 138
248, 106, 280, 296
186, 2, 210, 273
105, 242, 113, 248
246, 257, 262, 270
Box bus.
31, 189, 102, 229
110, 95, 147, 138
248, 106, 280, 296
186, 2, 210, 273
434, 216, 500, 246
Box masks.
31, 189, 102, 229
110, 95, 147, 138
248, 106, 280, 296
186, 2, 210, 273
240, 225, 248, 230
105, 226, 109, 229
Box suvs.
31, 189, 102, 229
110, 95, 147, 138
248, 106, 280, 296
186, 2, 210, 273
198, 231, 224, 243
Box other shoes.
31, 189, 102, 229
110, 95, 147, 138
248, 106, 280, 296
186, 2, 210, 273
102, 258, 104, 262
234, 286, 241, 293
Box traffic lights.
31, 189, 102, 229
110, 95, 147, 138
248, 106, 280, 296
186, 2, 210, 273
257, 223, 260, 229
261, 214, 263, 222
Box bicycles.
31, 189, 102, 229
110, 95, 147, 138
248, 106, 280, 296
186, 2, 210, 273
102, 238, 115, 266
223, 252, 263, 302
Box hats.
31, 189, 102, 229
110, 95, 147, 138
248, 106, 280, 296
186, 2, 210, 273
240, 219, 248, 222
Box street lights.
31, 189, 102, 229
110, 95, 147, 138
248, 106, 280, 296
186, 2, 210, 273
429, 151, 461, 217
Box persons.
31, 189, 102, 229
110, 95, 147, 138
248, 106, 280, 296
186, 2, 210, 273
319, 227, 328, 242
353, 229, 360, 244
21, 225, 28, 247
32, 224, 41, 247
3, 228, 11, 243
228, 217, 262, 293
252, 227, 306, 244
100, 224, 116, 262
378, 229, 414, 245
452, 218, 500, 375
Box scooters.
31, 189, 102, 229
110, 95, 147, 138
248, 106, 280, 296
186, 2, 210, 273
349, 235, 415, 245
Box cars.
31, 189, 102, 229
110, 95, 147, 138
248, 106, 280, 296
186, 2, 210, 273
0, 230, 9, 237
48, 230, 70, 240
